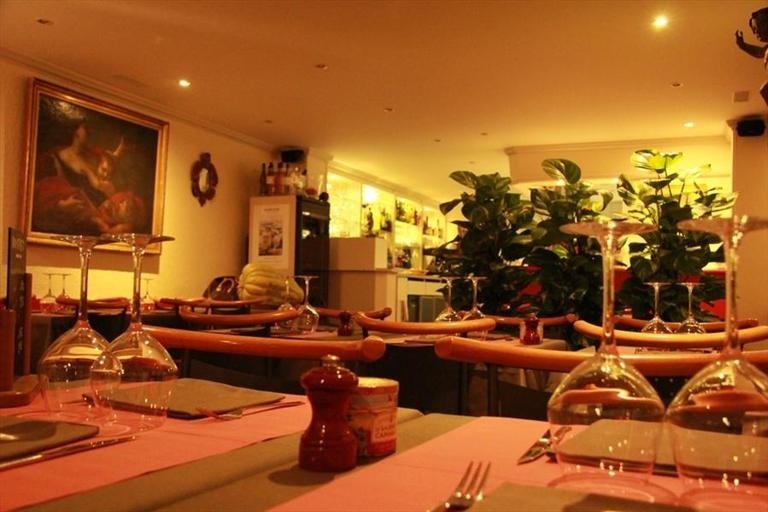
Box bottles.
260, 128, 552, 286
522, 316, 539, 344
258, 161, 303, 195
336, 309, 352, 336
295, 351, 360, 472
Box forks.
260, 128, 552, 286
193, 401, 302, 422
432, 453, 493, 512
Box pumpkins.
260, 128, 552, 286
238, 263, 304, 304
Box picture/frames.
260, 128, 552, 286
19, 75, 171, 256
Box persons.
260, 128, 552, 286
40, 122, 146, 233
734, 7, 767, 110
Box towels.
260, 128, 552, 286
0, 417, 100, 471
553, 417, 768, 482
460, 478, 693, 511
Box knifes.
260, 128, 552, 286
521, 419, 570, 467
0, 434, 136, 473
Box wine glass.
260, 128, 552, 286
462, 273, 491, 340
293, 275, 321, 335
54, 271, 71, 313
672, 279, 707, 349
547, 218, 666, 490
39, 232, 126, 424
40, 271, 56, 313
92, 232, 181, 440
273, 272, 298, 331
637, 281, 672, 351
435, 273, 462, 337
665, 217, 767, 511
140, 275, 155, 312
